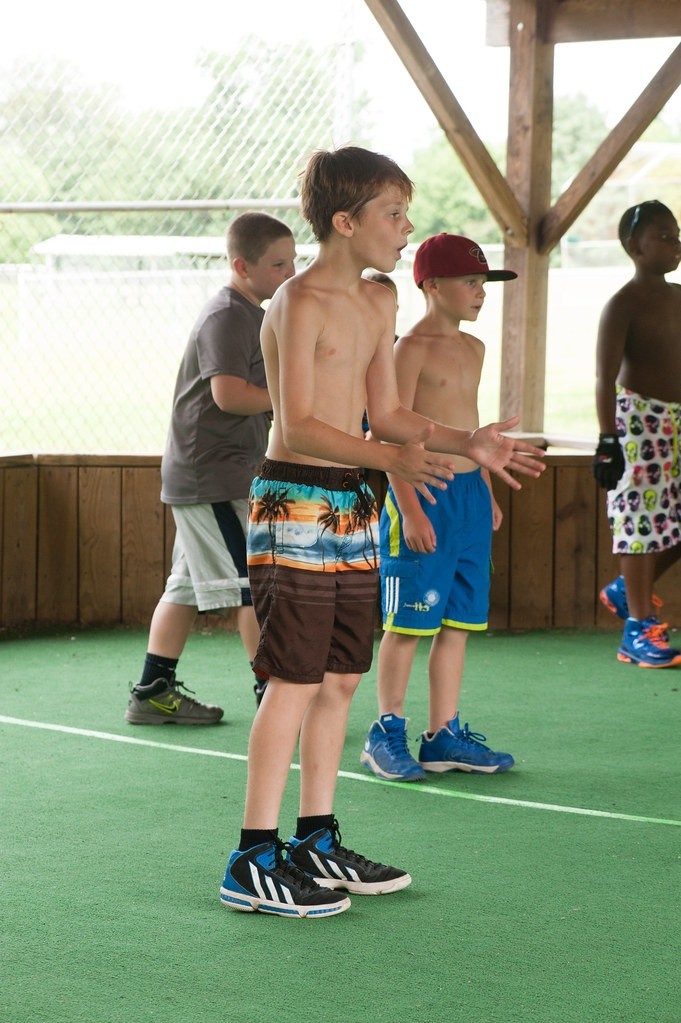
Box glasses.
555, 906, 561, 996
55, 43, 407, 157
627, 198, 667, 239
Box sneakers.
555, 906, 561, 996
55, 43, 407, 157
596, 575, 662, 626
214, 840, 351, 921
615, 617, 681, 670
354, 712, 426, 782
283, 825, 413, 896
125, 677, 225, 727
413, 710, 515, 776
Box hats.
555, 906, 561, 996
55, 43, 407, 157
412, 232, 519, 292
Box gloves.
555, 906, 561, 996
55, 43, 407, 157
592, 432, 626, 493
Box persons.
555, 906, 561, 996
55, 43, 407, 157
359, 231, 515, 782
126, 211, 297, 725
220, 150, 547, 918
360, 272, 400, 346
596, 199, 681, 669
